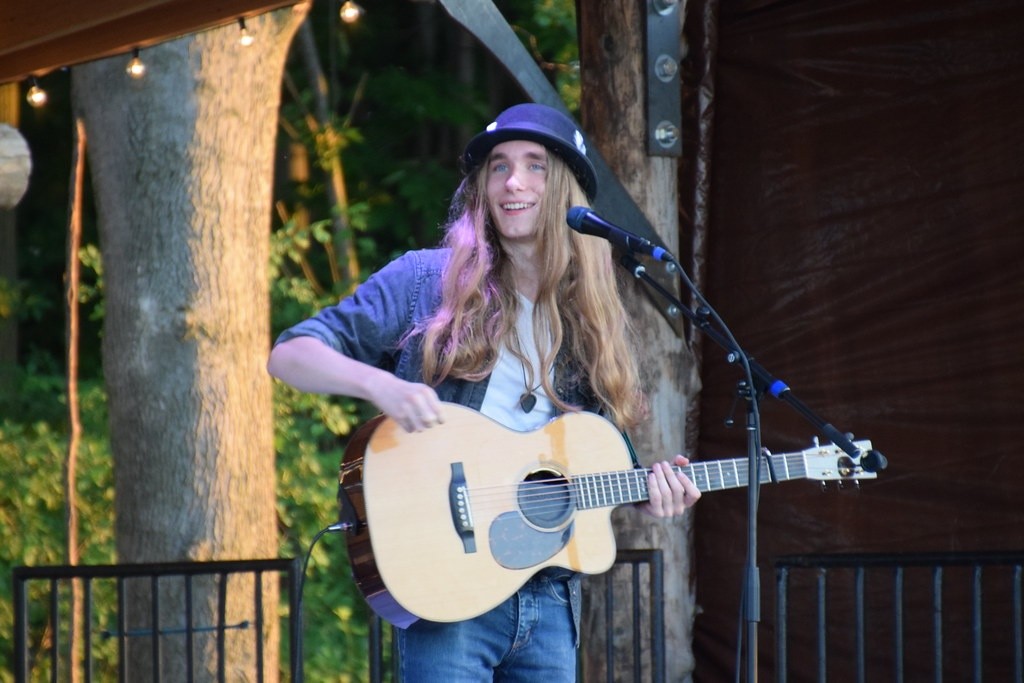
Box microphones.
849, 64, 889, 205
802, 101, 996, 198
566, 208, 676, 267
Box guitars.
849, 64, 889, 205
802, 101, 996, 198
339, 397, 892, 634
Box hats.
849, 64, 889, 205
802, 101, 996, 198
463, 103, 598, 204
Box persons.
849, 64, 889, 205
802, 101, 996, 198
270, 100, 704, 682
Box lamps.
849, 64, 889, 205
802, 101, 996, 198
239, 18, 254, 46
339, 0, 359, 23
27, 75, 47, 108
125, 50, 147, 79
